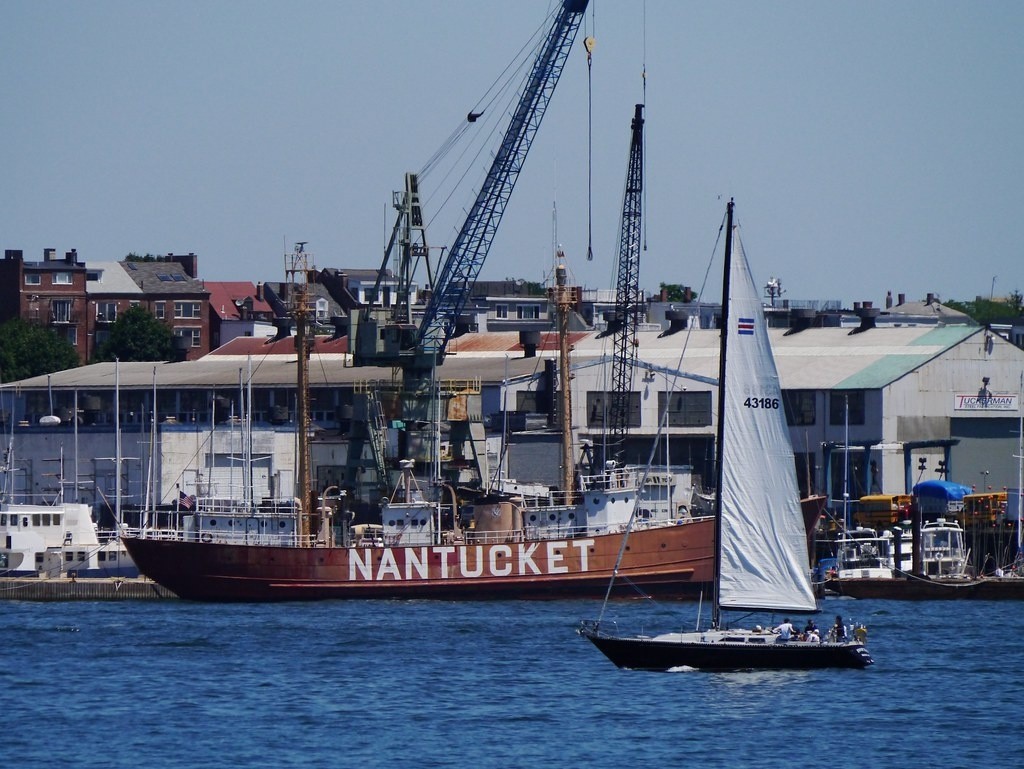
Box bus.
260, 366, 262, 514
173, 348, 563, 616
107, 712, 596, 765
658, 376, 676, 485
821, 481, 1024, 526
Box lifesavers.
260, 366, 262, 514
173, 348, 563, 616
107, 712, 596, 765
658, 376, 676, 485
201, 531, 213, 542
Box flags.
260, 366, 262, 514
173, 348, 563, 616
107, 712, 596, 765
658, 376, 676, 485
179, 491, 194, 509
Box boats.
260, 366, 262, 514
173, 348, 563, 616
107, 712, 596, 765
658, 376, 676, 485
111, 457, 718, 601
820, 519, 976, 591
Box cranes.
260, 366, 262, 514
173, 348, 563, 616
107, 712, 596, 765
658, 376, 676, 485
335, 2, 653, 510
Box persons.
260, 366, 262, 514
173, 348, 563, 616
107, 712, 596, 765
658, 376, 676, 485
804, 619, 817, 633
774, 618, 797, 644
828, 615, 848, 642
805, 628, 820, 642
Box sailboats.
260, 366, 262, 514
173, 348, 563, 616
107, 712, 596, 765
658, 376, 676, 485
2, 356, 291, 579
576, 193, 877, 673
